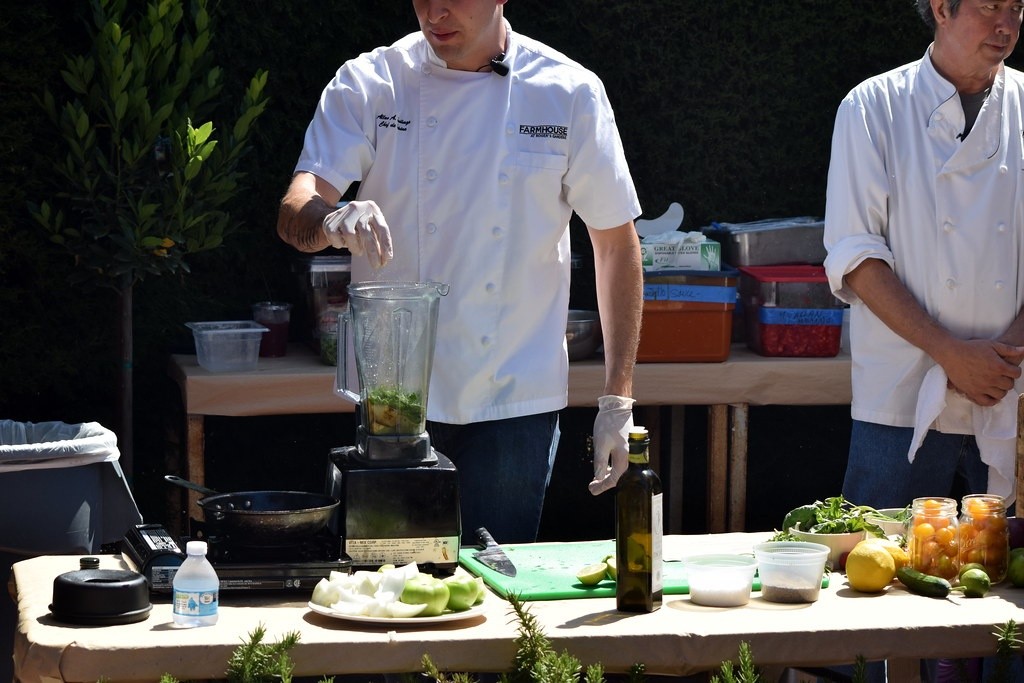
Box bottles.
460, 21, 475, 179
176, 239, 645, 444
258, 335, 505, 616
612, 429, 663, 614
170, 540, 220, 627
905, 496, 961, 586
958, 494, 1010, 585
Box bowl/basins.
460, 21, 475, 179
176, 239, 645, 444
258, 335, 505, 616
864, 507, 914, 540
184, 319, 271, 373
788, 523, 867, 569
682, 553, 759, 608
753, 540, 831, 603
564, 309, 602, 361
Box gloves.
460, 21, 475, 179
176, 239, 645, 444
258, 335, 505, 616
322, 199, 394, 270
589, 395, 634, 495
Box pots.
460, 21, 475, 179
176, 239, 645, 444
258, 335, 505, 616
164, 473, 335, 535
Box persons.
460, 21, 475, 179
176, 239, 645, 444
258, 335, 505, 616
278, 0, 642, 546
822, 0, 1024, 683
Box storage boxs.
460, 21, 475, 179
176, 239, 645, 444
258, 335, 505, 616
704, 221, 829, 266
310, 254, 351, 366
642, 240, 721, 271
742, 307, 846, 357
738, 266, 839, 305
634, 271, 740, 362
185, 321, 270, 372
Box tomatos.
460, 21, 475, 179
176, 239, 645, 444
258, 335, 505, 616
910, 500, 1008, 583
401, 574, 479, 615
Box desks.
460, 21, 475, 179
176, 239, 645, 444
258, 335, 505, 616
167, 352, 729, 542
12, 545, 530, 683
511, 528, 1024, 683
729, 340, 852, 538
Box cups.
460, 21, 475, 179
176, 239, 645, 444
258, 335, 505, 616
253, 299, 292, 359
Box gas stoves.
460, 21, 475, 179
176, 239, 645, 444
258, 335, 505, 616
124, 521, 354, 597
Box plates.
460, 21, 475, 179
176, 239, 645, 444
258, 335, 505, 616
307, 599, 485, 623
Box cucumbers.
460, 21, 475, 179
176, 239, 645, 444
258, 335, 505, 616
896, 566, 952, 598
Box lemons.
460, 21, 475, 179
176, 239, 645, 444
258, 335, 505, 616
958, 547, 1024, 597
845, 538, 907, 592
576, 557, 617, 586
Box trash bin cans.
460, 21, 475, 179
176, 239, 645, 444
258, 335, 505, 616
0, 418, 143, 578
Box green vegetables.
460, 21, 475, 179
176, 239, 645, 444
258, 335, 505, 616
765, 494, 911, 549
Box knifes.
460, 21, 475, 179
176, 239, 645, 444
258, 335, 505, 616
473, 526, 517, 579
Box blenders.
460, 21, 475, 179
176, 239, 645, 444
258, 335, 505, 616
332, 278, 462, 579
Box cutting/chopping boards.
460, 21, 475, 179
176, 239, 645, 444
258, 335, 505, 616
458, 538, 761, 600
